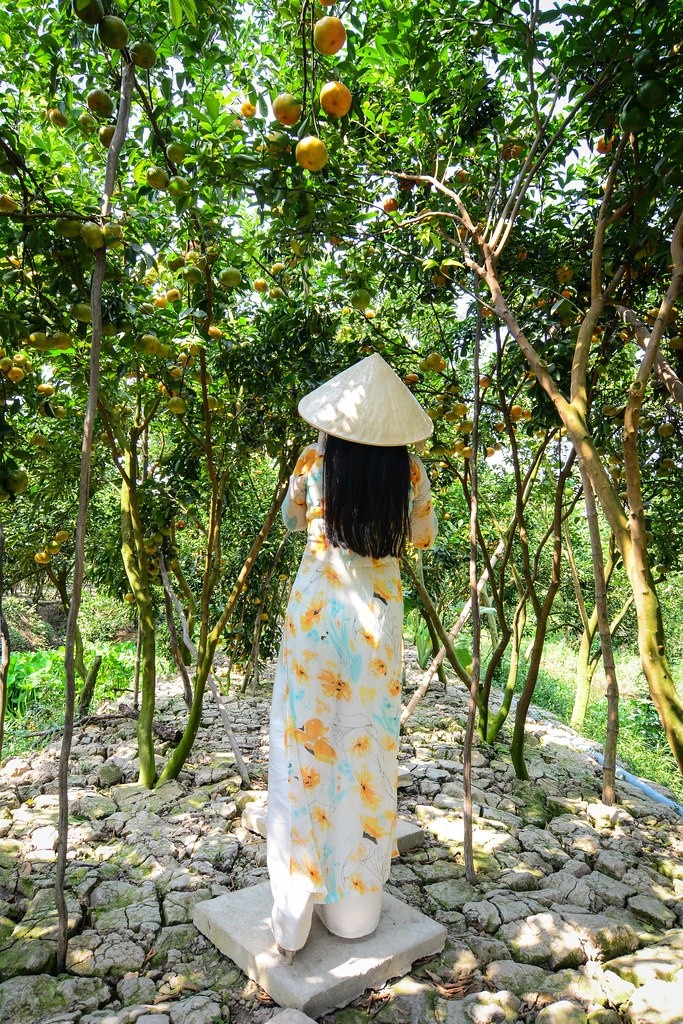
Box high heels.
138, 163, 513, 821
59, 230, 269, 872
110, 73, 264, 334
276, 942, 298, 965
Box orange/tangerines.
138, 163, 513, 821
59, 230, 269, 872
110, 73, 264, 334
0, 0, 683, 678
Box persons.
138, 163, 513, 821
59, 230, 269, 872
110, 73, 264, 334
265, 354, 438, 968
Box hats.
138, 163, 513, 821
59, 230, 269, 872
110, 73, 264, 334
297, 352, 434, 446
